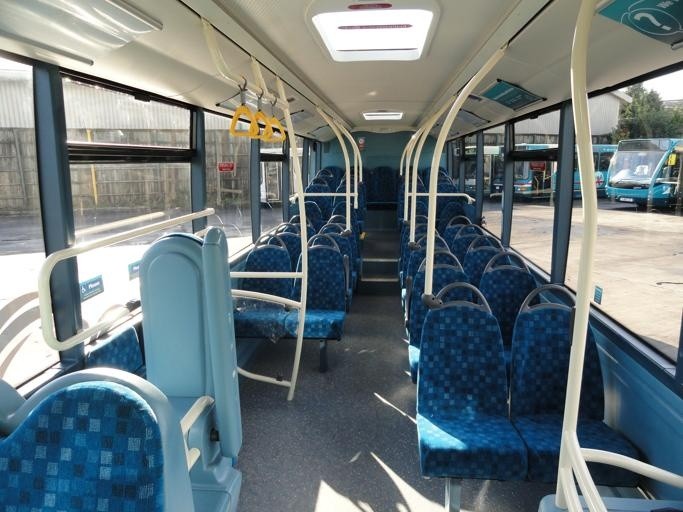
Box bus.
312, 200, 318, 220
259, 147, 310, 207
490, 143, 620, 200
452, 146, 502, 198
605, 136, 683, 211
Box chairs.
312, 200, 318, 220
369, 164, 399, 211
1, 364, 196, 512
413, 279, 531, 510
509, 281, 646, 485
398, 166, 546, 386
231, 164, 369, 375
